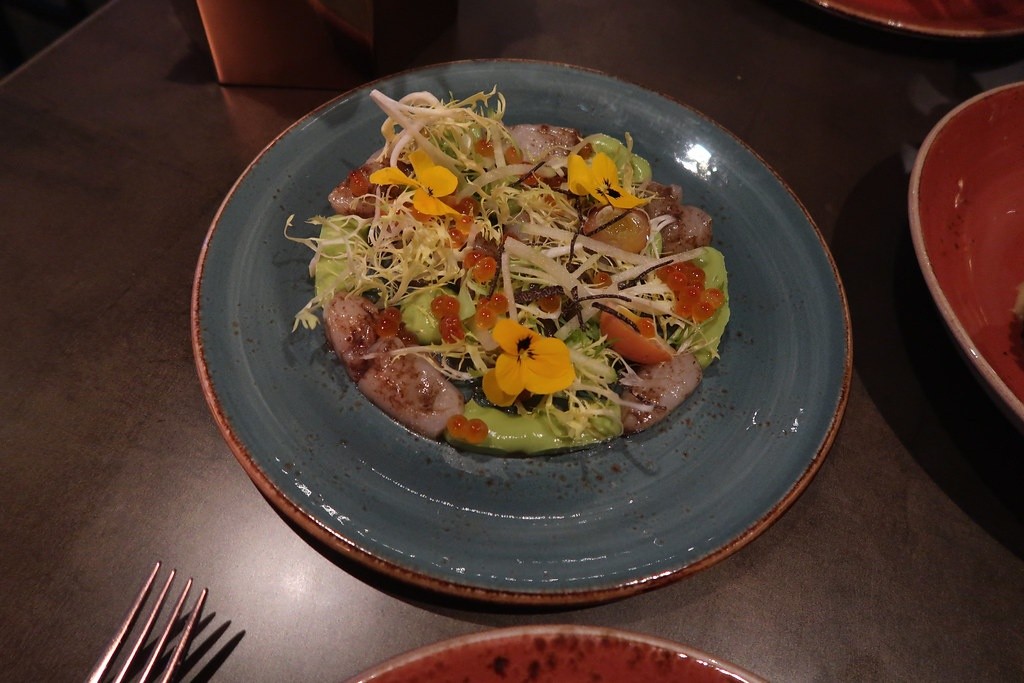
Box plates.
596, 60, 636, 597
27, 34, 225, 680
907, 83, 1024, 417
817, 0, 1024, 43
189, 56, 854, 604
342, 625, 771, 683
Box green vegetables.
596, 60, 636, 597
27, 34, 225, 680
283, 87, 717, 440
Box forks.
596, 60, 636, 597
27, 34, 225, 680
84, 561, 208, 683
